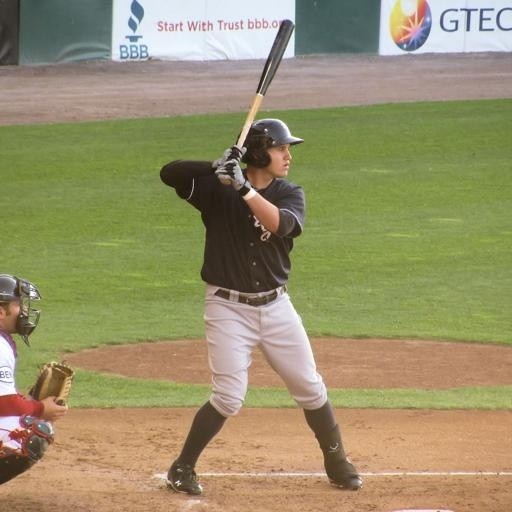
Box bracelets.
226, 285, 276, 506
242, 188, 257, 200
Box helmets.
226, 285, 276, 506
236, 118, 305, 168
0, 273, 43, 330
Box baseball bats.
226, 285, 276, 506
224, 19, 295, 177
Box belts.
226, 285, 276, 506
215, 285, 286, 307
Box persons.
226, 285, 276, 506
0, 272, 70, 486
159, 113, 363, 498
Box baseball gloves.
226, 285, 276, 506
28, 358, 74, 408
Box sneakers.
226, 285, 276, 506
324, 457, 362, 490
167, 459, 203, 495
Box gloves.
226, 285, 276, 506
210, 144, 247, 167
214, 160, 251, 196
